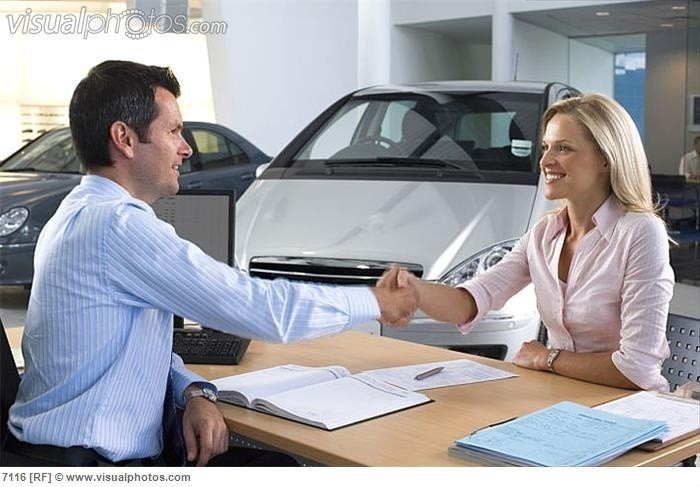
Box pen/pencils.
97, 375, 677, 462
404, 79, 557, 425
469, 415, 520, 436
415, 366, 444, 381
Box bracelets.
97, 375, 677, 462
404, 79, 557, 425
546, 347, 562, 375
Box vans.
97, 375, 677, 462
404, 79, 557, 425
230, 76, 586, 364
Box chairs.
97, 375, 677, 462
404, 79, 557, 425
389, 107, 448, 156
500, 111, 536, 172
661, 280, 700, 400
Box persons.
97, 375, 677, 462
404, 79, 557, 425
370, 90, 676, 395
1, 58, 422, 464
678, 135, 700, 182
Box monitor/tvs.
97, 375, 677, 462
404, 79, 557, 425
157, 188, 236, 327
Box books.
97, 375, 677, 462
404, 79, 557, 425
206, 362, 435, 431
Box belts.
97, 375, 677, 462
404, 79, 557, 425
6, 432, 97, 466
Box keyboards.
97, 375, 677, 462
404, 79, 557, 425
170, 327, 251, 366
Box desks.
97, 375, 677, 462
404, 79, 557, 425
1, 325, 700, 465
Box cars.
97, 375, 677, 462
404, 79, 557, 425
1, 120, 276, 293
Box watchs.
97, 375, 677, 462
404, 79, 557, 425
182, 386, 218, 403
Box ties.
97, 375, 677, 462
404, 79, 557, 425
165, 356, 187, 466
